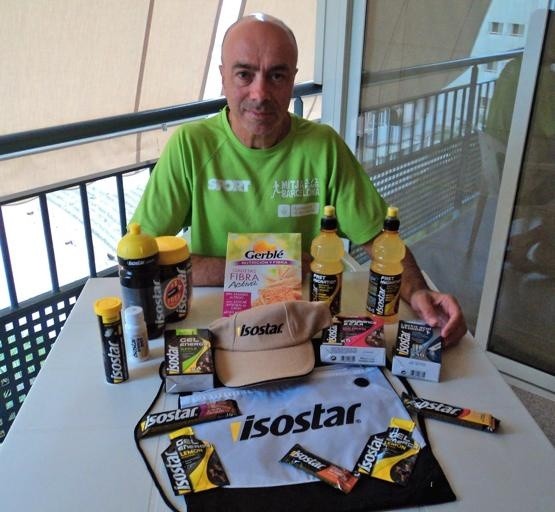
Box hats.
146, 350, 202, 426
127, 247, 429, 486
208, 301, 332, 387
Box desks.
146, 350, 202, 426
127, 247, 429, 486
0, 269, 555, 512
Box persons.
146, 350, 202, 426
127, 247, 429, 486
127, 13, 468, 351
484, 9, 555, 275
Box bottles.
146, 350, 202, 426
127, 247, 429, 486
308, 206, 406, 325
95, 225, 191, 383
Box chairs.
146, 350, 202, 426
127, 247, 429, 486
342, 251, 362, 272
466, 130, 555, 271
